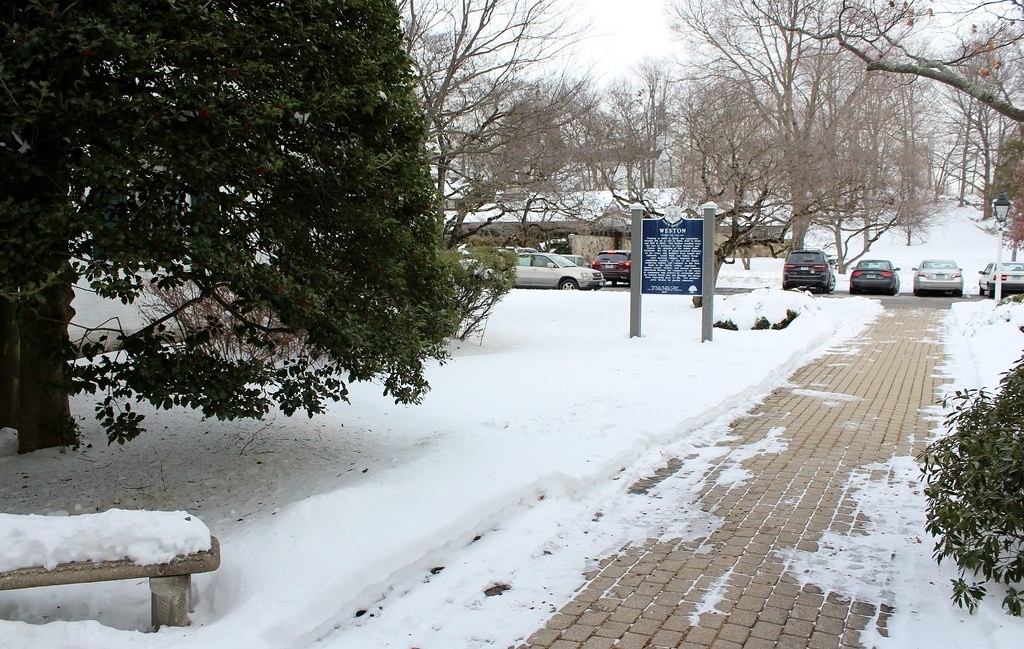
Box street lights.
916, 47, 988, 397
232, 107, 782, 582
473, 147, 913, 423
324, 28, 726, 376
993, 190, 1012, 304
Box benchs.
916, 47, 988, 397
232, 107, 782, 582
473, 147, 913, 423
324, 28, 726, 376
0, 534, 221, 633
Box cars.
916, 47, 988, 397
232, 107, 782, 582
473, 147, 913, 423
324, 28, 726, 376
912, 259, 965, 297
847, 259, 901, 295
978, 260, 1024, 297
455, 244, 542, 283
510, 253, 606, 291
561, 254, 590, 266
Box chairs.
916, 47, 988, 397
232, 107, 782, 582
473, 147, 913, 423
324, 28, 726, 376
787, 256, 1024, 272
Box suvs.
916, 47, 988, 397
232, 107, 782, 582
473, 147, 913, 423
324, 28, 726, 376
782, 248, 837, 295
592, 249, 631, 286
